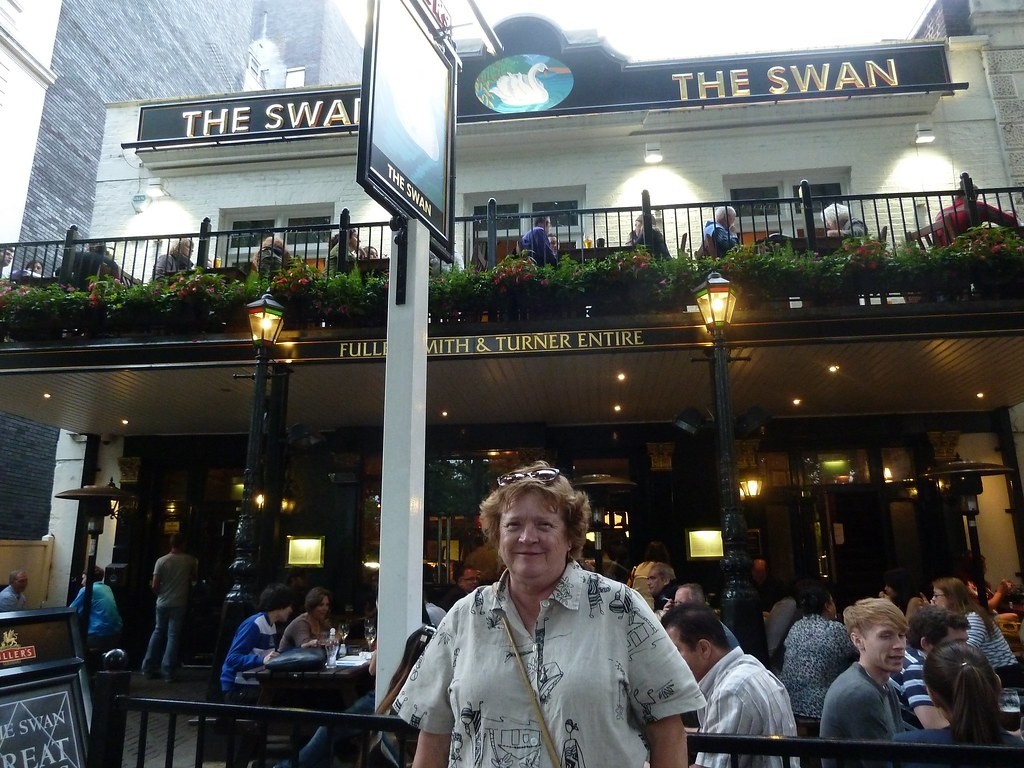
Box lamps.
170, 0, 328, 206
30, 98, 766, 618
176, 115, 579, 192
675, 406, 707, 437
914, 121, 936, 145
733, 405, 773, 443
148, 177, 165, 199
643, 141, 663, 164
288, 422, 327, 450
739, 465, 763, 502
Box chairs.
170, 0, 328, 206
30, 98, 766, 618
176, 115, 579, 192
858, 224, 889, 304
681, 232, 688, 251
97, 264, 118, 281
694, 234, 719, 260
905, 214, 958, 302
117, 267, 143, 291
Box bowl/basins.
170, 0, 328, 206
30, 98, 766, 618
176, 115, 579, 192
815, 228, 831, 236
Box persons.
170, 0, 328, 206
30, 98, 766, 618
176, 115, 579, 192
935, 184, 1018, 246
69, 566, 124, 646
645, 560, 800, 768
0, 569, 28, 611
26, 260, 44, 275
705, 206, 739, 259
512, 216, 560, 269
440, 565, 479, 612
393, 461, 708, 768
282, 566, 308, 611
820, 203, 868, 238
252, 236, 291, 279
630, 213, 673, 263
323, 229, 378, 276
219, 584, 447, 768
781, 578, 1024, 768
0, 246, 14, 280
141, 534, 197, 682
149, 240, 194, 283
963, 550, 1020, 629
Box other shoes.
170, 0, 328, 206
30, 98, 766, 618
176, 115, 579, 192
144, 666, 194, 682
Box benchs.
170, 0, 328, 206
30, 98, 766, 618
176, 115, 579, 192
188, 716, 260, 765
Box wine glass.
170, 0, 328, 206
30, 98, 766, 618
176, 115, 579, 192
364, 627, 377, 651
338, 623, 350, 644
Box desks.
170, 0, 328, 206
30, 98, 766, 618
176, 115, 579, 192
236, 639, 371, 768
556, 245, 636, 264
756, 236, 845, 310
161, 266, 248, 284
21, 276, 60, 288
344, 258, 389, 276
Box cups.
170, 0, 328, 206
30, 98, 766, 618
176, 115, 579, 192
214, 255, 221, 267
797, 227, 804, 238
997, 688, 1021, 732
584, 232, 592, 248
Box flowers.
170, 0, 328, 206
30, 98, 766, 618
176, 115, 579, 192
0, 221, 1024, 345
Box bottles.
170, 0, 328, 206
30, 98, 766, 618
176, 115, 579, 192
325, 627, 339, 668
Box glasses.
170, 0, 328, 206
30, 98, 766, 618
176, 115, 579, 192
932, 594, 946, 598
495, 467, 562, 486
